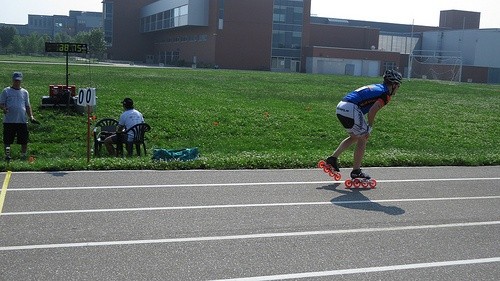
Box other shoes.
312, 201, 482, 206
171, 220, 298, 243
5, 156, 11, 163
21, 153, 27, 160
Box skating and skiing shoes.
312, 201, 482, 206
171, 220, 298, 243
345, 168, 377, 189
318, 156, 342, 181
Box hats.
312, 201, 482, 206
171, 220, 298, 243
120, 98, 133, 105
12, 72, 23, 81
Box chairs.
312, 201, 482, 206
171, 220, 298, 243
93, 118, 124, 158
115, 123, 151, 158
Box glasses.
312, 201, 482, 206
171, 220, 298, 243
395, 83, 400, 88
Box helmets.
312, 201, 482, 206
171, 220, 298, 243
383, 69, 402, 86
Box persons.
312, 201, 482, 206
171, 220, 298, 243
0, 72, 40, 161
318, 70, 403, 189
104, 98, 144, 158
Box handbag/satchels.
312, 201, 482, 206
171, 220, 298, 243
152, 148, 200, 164
